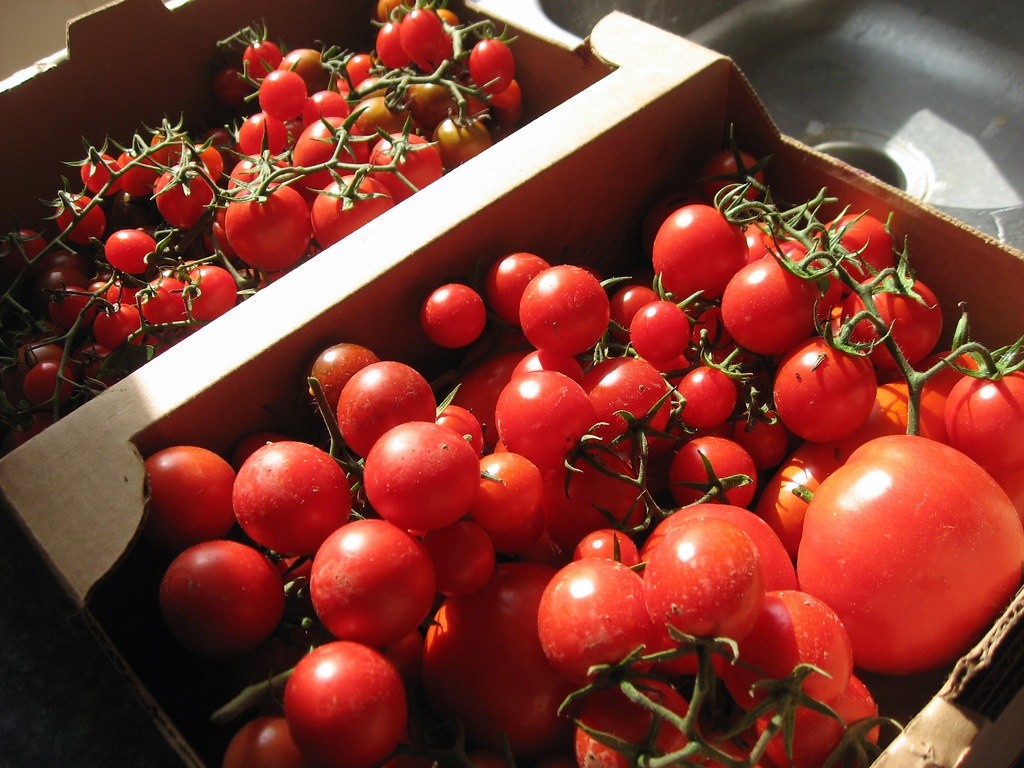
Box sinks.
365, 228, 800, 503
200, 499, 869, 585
535, 1, 1024, 259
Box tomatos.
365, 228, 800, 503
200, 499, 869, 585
0, 0, 521, 451
123, 140, 1023, 767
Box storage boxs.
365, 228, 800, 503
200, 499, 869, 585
0, 1, 1024, 768
